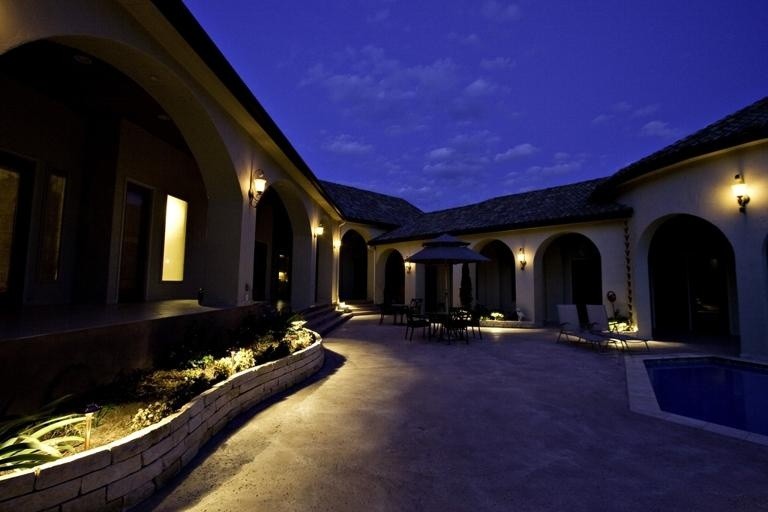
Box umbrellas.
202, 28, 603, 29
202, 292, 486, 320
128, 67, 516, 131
405, 232, 492, 311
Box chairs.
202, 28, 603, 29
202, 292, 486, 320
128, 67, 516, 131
554, 305, 651, 357
377, 298, 483, 346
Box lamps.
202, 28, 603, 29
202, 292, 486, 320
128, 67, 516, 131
404, 254, 412, 273
248, 168, 267, 199
518, 248, 527, 270
732, 172, 751, 219
315, 222, 324, 238
